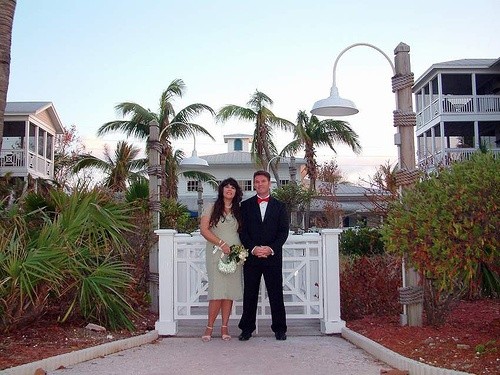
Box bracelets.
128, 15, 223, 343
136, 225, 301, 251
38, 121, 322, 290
212, 240, 226, 254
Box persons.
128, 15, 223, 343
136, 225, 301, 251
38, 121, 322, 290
201, 177, 243, 342
238, 171, 290, 341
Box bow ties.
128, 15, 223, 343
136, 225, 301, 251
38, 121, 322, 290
257, 197, 269, 204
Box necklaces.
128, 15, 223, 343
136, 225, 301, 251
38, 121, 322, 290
225, 204, 232, 208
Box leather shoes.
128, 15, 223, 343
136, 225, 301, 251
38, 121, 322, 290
238, 330, 255, 341
274, 333, 286, 340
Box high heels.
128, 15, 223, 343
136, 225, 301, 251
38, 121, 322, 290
201, 326, 213, 342
221, 325, 231, 341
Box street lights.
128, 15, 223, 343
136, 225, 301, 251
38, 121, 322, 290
265, 156, 291, 183
157, 122, 210, 229
310, 43, 407, 314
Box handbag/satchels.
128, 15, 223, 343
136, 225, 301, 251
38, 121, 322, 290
216, 251, 237, 275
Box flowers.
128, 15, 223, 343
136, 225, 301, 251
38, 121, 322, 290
216, 244, 249, 275
